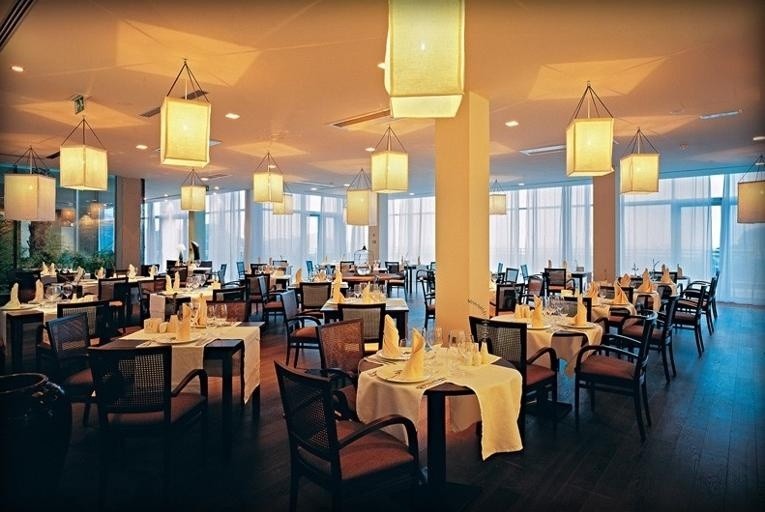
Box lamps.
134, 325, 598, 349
489, 181, 509, 215
60, 119, 107, 192
737, 156, 765, 224
619, 129, 660, 193
566, 86, 614, 177
4, 148, 56, 223
371, 127, 409, 194
253, 152, 283, 204
346, 169, 378, 226
180, 169, 207, 211
160, 63, 211, 168
389, 1, 465, 121
273, 180, 294, 216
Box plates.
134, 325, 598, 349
557, 320, 594, 328
526, 323, 552, 329
158, 331, 201, 343
376, 364, 431, 383
378, 346, 434, 360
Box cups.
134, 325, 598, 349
215, 304, 227, 338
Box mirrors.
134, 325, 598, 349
12, 165, 118, 272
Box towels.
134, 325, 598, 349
318, 271, 325, 281
335, 269, 342, 282
361, 284, 371, 303
333, 279, 344, 303
530, 295, 545, 327
397, 327, 426, 380
173, 271, 180, 290
677, 264, 682, 277
34, 279, 44, 302
199, 293, 207, 321
181, 303, 190, 338
5, 283, 19, 307
661, 268, 669, 283
621, 274, 631, 287
130, 264, 135, 279
165, 274, 172, 292
612, 283, 628, 304
383, 314, 401, 357
49, 263, 54, 275
43, 262, 48, 274
638, 269, 653, 294
588, 282, 597, 304
574, 293, 587, 325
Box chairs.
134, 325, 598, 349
420, 277, 435, 330
206, 300, 251, 322
549, 296, 609, 356
154, 275, 175, 293
273, 359, 419, 511
257, 276, 288, 323
244, 274, 270, 322
492, 263, 503, 278
218, 270, 225, 285
575, 312, 658, 428
468, 316, 558, 453
299, 282, 332, 312
649, 271, 678, 285
46, 312, 94, 425
194, 261, 212, 274
427, 271, 435, 305
598, 286, 634, 327
138, 279, 166, 318
430, 262, 436, 271
212, 287, 244, 301
272, 260, 287, 267
250, 264, 267, 274
520, 264, 530, 294
280, 290, 324, 369
674, 277, 717, 336
237, 262, 245, 278
87, 345, 209, 483
337, 303, 386, 355
658, 283, 708, 357
617, 296, 679, 384
501, 267, 519, 284
522, 275, 544, 311
168, 265, 188, 282
141, 264, 159, 277
306, 261, 313, 272
495, 283, 524, 316
685, 270, 720, 320
385, 262, 399, 273
315, 318, 365, 420
221, 263, 227, 275
167, 260, 183, 274
389, 264, 409, 301
98, 277, 129, 300
618, 277, 643, 289
340, 261, 354, 273
34, 299, 110, 371
544, 268, 576, 295
